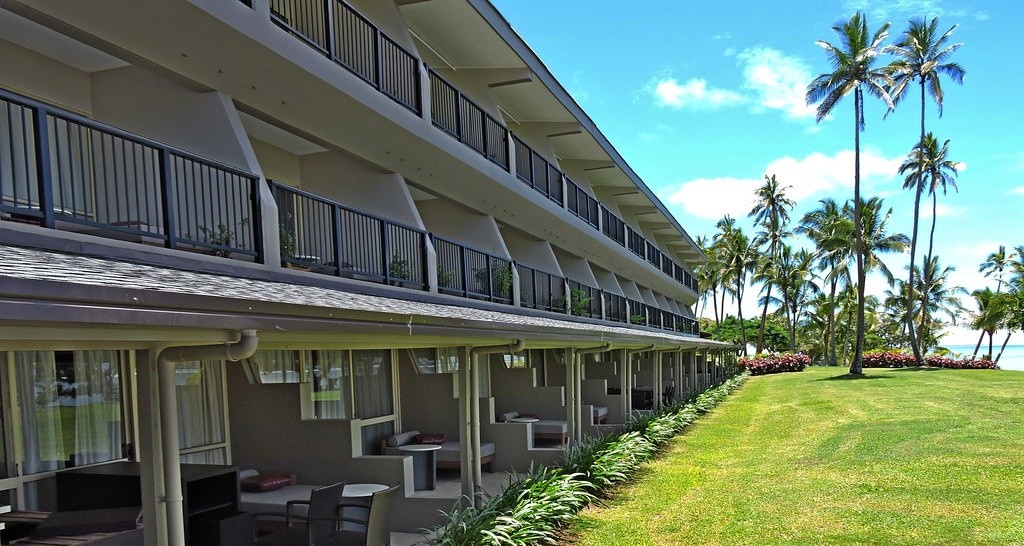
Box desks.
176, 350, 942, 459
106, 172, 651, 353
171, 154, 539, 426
10, 205, 73, 226
509, 418, 539, 448
342, 484, 390, 532
292, 254, 320, 271
398, 444, 442, 491
633, 387, 653, 412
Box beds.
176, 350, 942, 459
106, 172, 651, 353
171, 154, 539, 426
240, 469, 343, 531
311, 261, 383, 283
381, 430, 496, 473
593, 406, 609, 425
499, 411, 568, 446
70, 220, 213, 255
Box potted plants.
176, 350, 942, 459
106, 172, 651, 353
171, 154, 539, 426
437, 264, 455, 292
280, 226, 294, 268
521, 292, 527, 307
492, 260, 510, 304
378, 250, 410, 287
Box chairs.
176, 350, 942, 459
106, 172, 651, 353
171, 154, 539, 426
662, 384, 671, 402
250, 480, 347, 546
616, 388, 622, 395
651, 386, 668, 408
314, 480, 401, 546
663, 387, 675, 406
632, 389, 650, 410
607, 387, 615, 395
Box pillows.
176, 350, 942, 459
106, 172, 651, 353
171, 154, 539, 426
240, 468, 259, 491
323, 261, 353, 269
516, 413, 544, 421
388, 430, 420, 447
250, 473, 292, 491
110, 221, 150, 227
499, 411, 519, 421
413, 433, 448, 444
